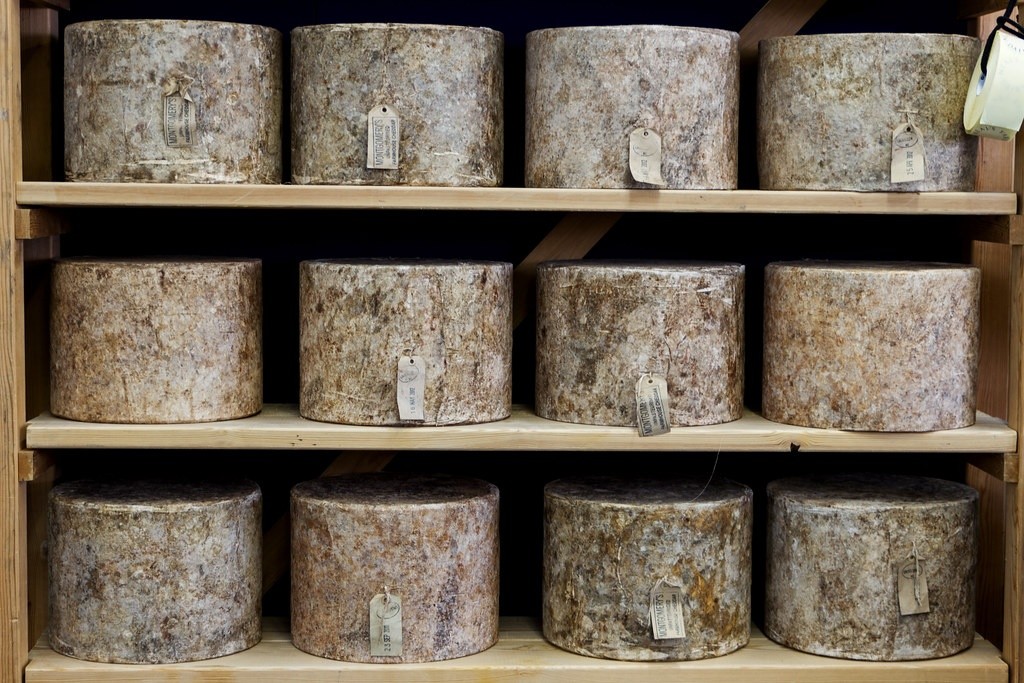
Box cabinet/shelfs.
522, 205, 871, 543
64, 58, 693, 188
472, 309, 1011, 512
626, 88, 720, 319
0, 182, 1023, 683
